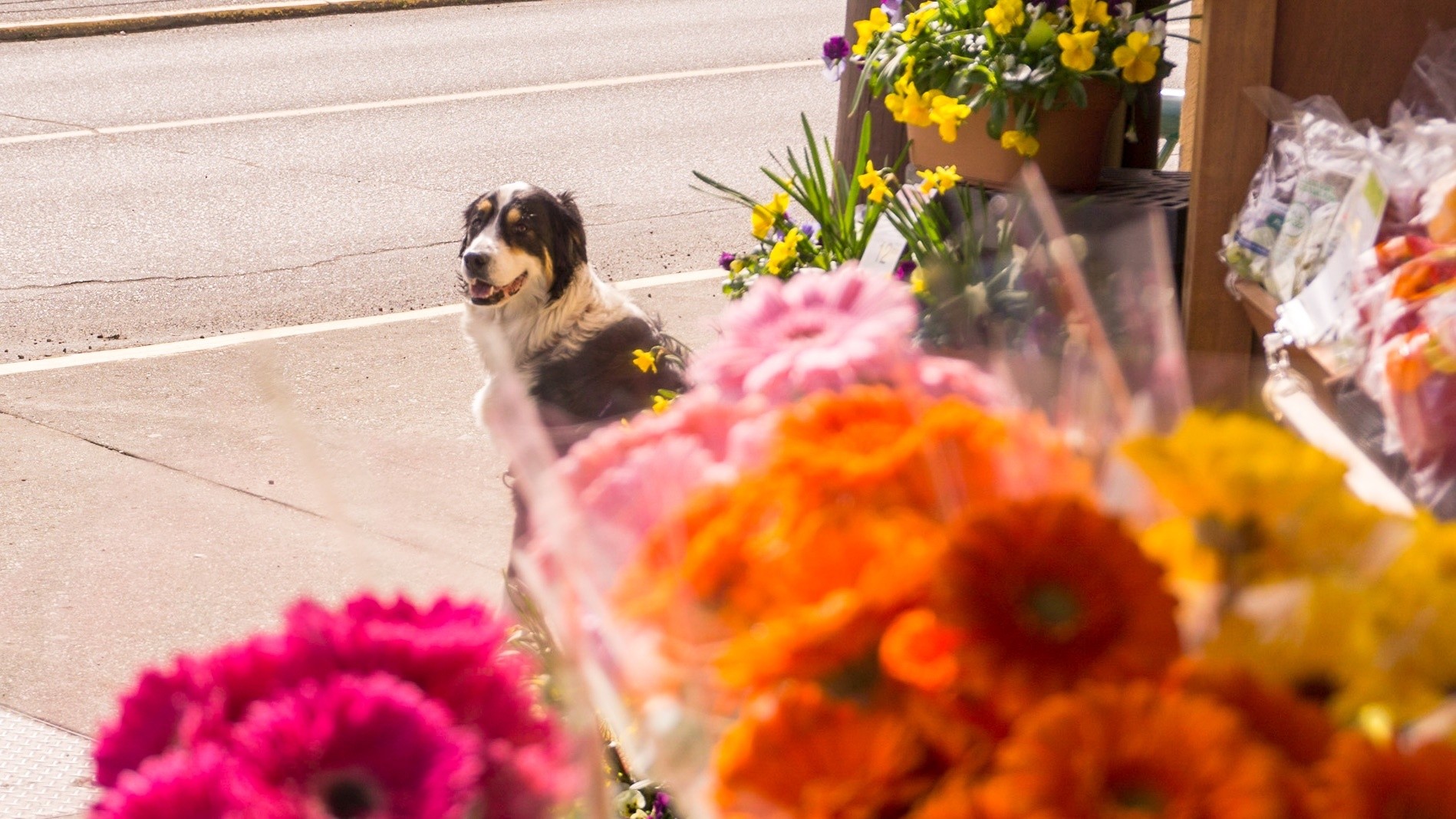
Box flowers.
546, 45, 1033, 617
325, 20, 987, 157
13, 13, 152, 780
82, 101, 1456, 819
821, 0, 1204, 158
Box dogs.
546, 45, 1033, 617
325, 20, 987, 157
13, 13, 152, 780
455, 180, 693, 620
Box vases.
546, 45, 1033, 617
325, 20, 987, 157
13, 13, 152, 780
906, 79, 1124, 194
